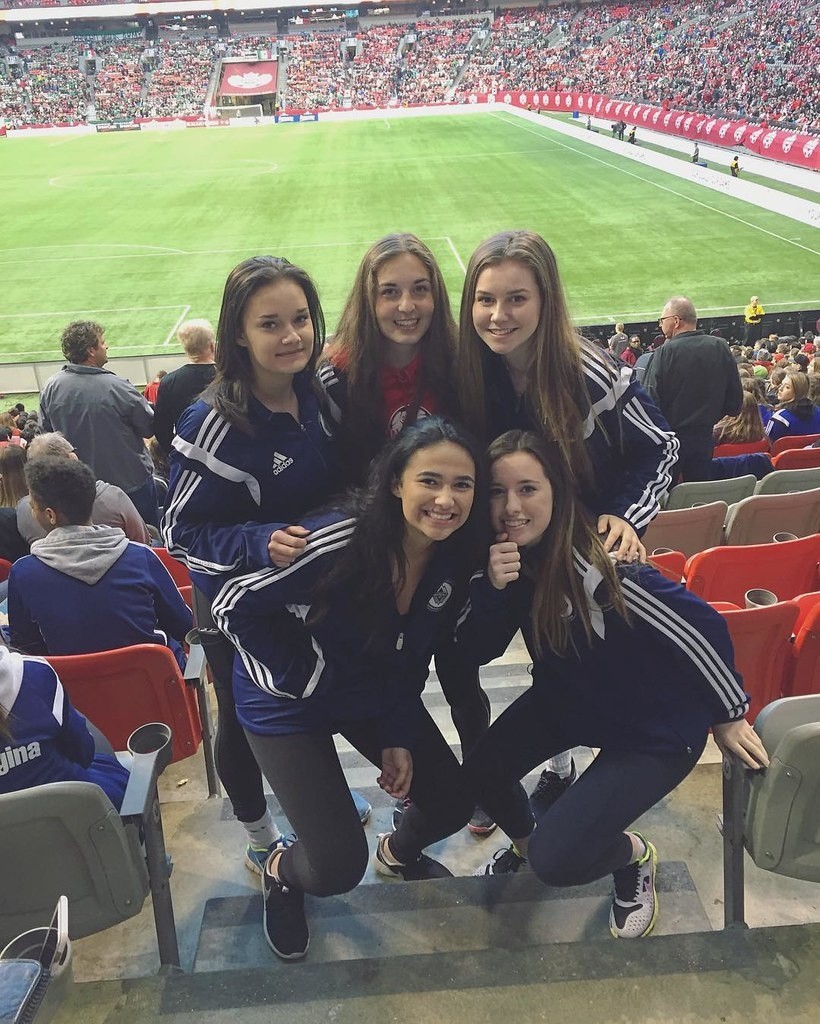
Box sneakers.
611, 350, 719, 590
392, 796, 413, 831
607, 830, 659, 938
261, 842, 310, 958
473, 842, 529, 876
350, 789, 372, 822
466, 805, 496, 832
373, 831, 454, 882
244, 833, 297, 875
528, 756, 580, 823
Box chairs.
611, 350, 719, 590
0, 432, 820, 1024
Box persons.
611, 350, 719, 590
144, 438, 170, 505
15, 434, 163, 548
457, 232, 687, 819
692, 143, 699, 162
591, 324, 666, 386
731, 156, 740, 177
643, 298, 745, 492
211, 415, 485, 960
450, 428, 769, 938
160, 255, 371, 879
144, 371, 168, 405
38, 322, 160, 530
316, 235, 458, 829
629, 126, 636, 144
0, 0, 820, 136
0, 642, 159, 843
153, 318, 221, 455
0, 403, 40, 456
713, 319, 819, 446
0, 442, 29, 647
745, 296, 765, 347
8, 455, 194, 671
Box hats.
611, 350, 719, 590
802, 343, 816, 351
790, 354, 808, 366
754, 365, 768, 378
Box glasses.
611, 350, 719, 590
71, 447, 79, 455
658, 315, 681, 326
